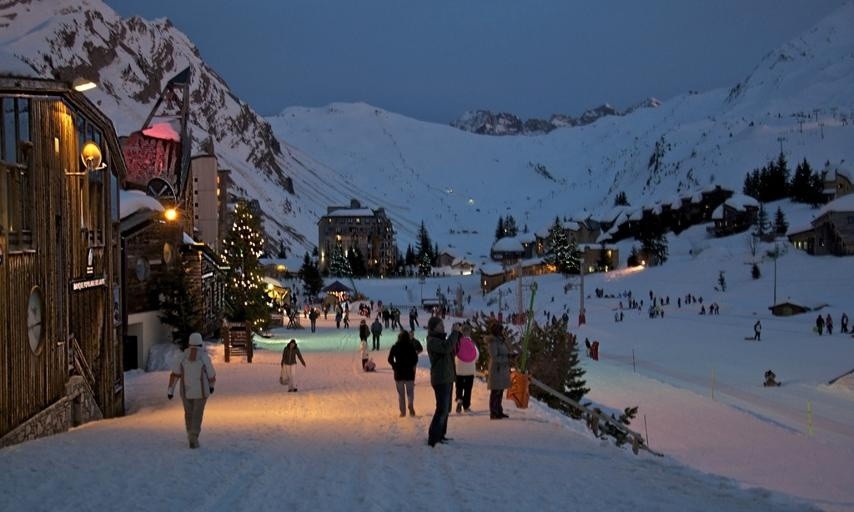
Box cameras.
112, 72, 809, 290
453, 322, 462, 327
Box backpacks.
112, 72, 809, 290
456, 338, 476, 362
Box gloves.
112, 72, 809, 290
210, 387, 213, 393
168, 395, 173, 399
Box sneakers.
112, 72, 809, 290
456, 403, 462, 413
491, 414, 508, 418
400, 404, 415, 417
288, 388, 297, 392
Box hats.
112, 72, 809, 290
428, 317, 441, 329
189, 333, 203, 346
458, 325, 472, 335
491, 323, 503, 336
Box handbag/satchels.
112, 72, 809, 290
280, 376, 287, 384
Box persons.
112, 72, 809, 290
762, 369, 781, 388
815, 313, 825, 336
595, 287, 721, 321
839, 310, 849, 333
825, 312, 834, 336
279, 338, 307, 395
752, 319, 762, 340
483, 321, 519, 420
423, 317, 461, 446
165, 331, 216, 448
301, 297, 421, 371
388, 328, 420, 419
448, 321, 479, 413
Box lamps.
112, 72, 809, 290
73, 75, 102, 175
